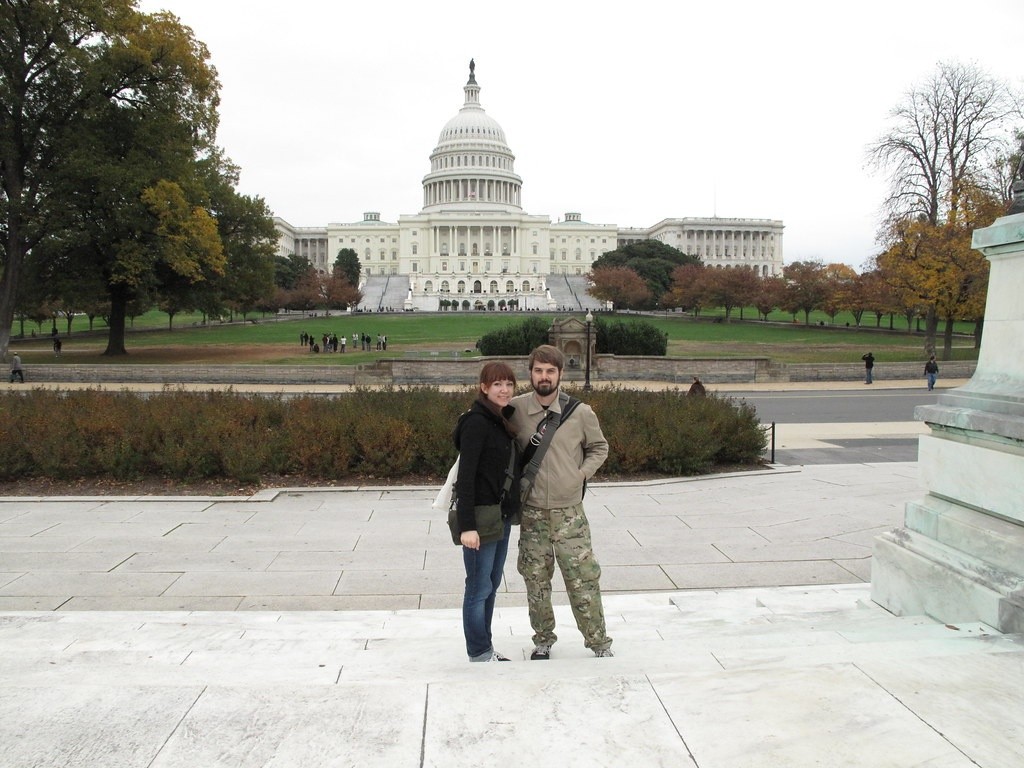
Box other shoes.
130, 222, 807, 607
531, 644, 551, 659
484, 654, 512, 663
593, 649, 615, 657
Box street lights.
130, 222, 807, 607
583, 310, 595, 390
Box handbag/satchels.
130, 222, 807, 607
434, 455, 463, 510
508, 475, 533, 525
446, 504, 506, 546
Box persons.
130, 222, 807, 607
383, 335, 387, 350
460, 342, 614, 656
477, 303, 617, 315
451, 361, 521, 661
309, 335, 315, 351
320, 331, 338, 354
9, 352, 24, 384
360, 332, 366, 350
340, 336, 347, 353
304, 332, 309, 346
351, 303, 407, 317
861, 352, 876, 385
306, 310, 334, 318
54, 337, 63, 358
366, 334, 371, 352
300, 331, 305, 346
313, 344, 320, 353
922, 355, 939, 390
352, 331, 359, 348
377, 333, 382, 350
